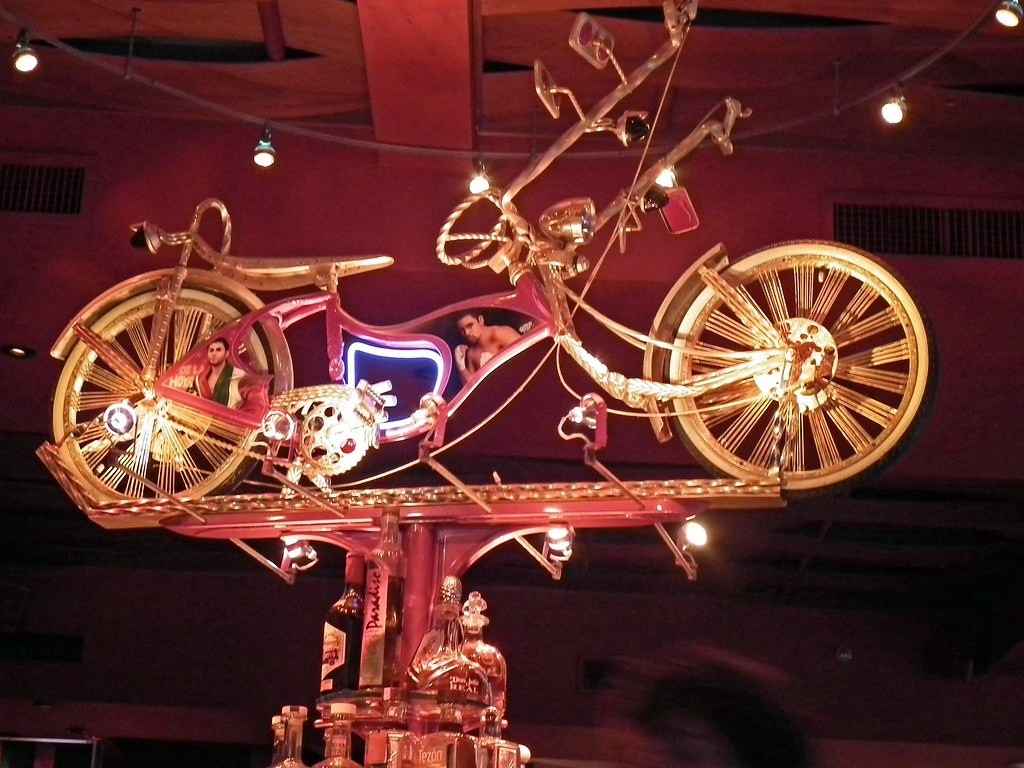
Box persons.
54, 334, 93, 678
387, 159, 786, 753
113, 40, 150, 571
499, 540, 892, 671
187, 337, 248, 408
455, 310, 522, 387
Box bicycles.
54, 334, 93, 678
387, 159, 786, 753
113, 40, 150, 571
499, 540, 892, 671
45, 0, 938, 507
